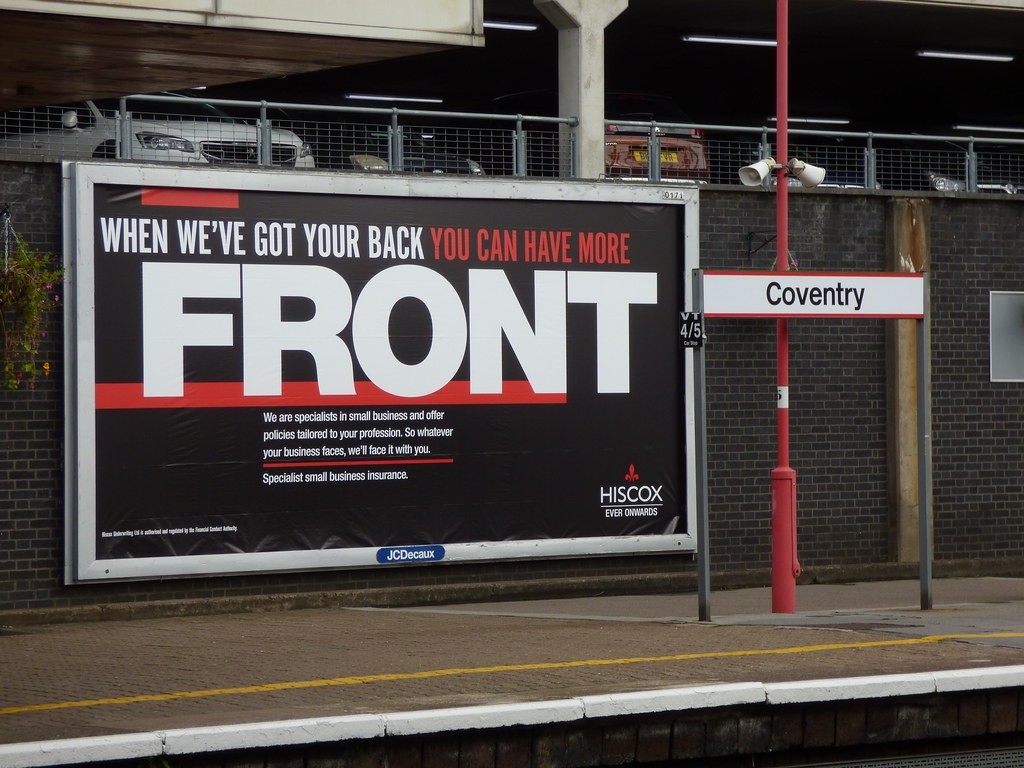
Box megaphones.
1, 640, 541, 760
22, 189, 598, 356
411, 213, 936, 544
788, 157, 826, 187
738, 157, 776, 187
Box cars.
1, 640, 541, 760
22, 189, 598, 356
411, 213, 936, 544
309, 126, 486, 177
475, 90, 710, 182
0, 91, 317, 168
